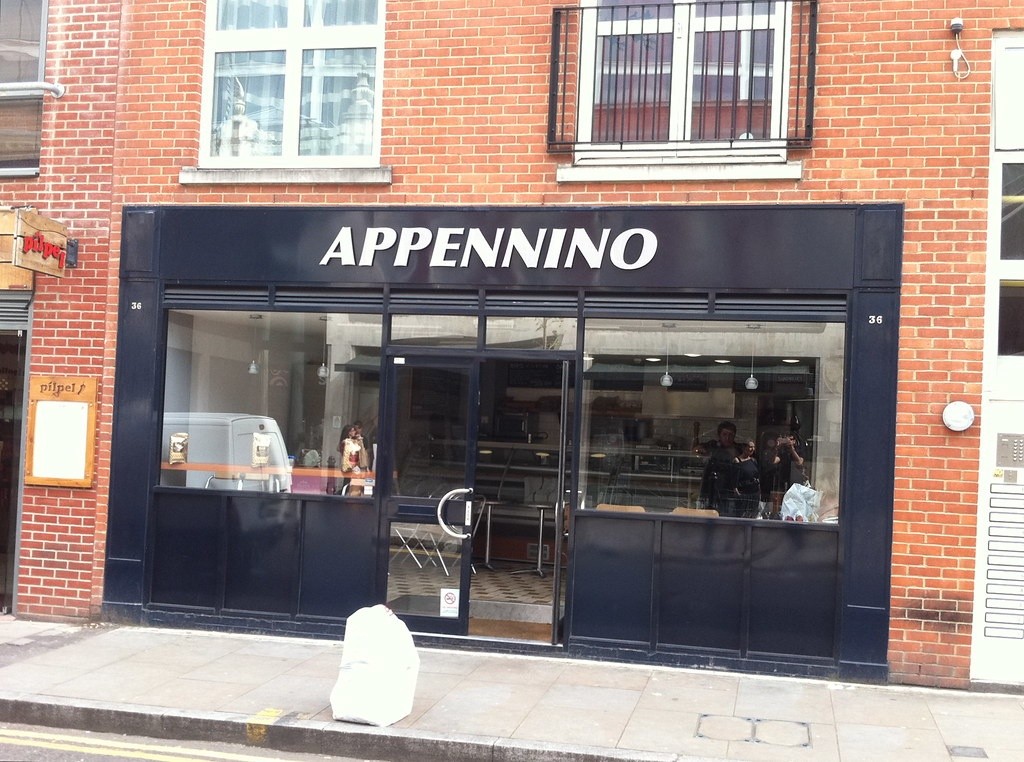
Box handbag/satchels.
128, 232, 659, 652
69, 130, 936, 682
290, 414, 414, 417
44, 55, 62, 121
756, 501, 764, 519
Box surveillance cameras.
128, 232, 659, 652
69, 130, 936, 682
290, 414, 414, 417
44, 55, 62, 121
949, 16, 964, 33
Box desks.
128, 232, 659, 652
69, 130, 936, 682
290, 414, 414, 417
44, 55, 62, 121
161, 461, 398, 495
469, 494, 566, 578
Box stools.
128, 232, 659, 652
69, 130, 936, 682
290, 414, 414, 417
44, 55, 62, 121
204, 472, 281, 493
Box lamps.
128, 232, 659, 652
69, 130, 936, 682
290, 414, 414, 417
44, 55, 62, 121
247, 313, 264, 374
660, 322, 676, 387
745, 324, 762, 389
317, 315, 333, 377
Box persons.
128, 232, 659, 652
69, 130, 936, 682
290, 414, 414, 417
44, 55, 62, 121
732, 432, 803, 518
337, 419, 368, 495
694, 422, 745, 512
